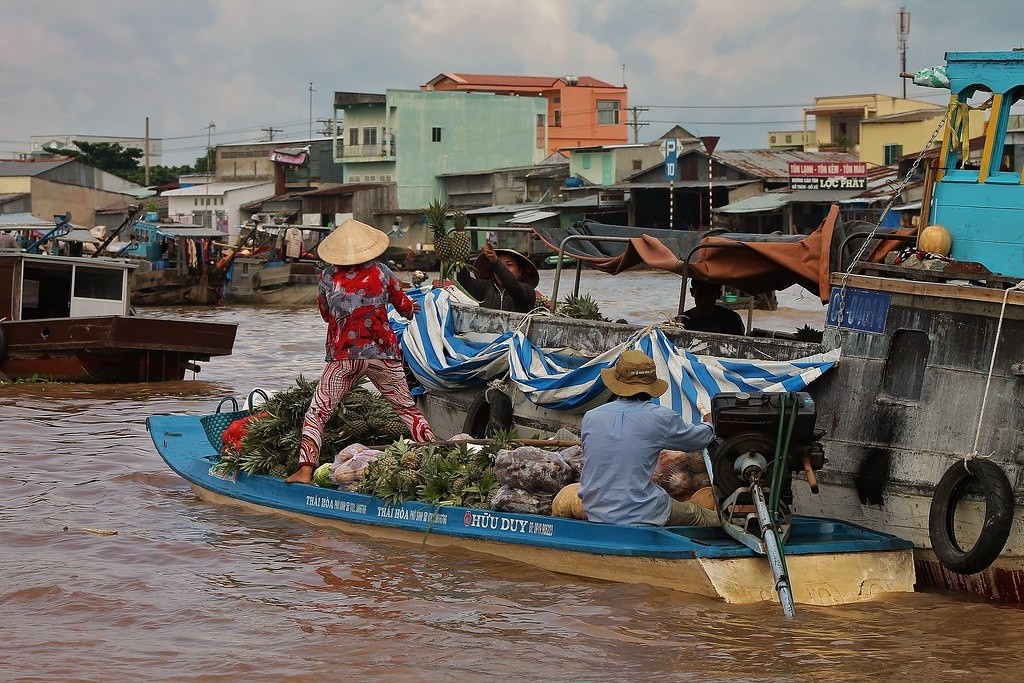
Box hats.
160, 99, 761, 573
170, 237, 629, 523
601, 351, 669, 398
474, 248, 539, 289
317, 218, 389, 265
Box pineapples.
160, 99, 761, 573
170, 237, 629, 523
362, 426, 550, 508
215, 372, 411, 477
422, 198, 470, 261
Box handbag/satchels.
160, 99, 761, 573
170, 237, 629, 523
200, 389, 269, 455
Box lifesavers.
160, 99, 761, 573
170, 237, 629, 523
251, 273, 261, 290
460, 389, 513, 439
927, 456, 1014, 573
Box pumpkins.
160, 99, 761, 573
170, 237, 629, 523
552, 482, 588, 520
919, 224, 952, 257
335, 445, 378, 483
652, 449, 718, 511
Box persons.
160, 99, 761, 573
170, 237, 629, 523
577, 350, 721, 529
284, 218, 438, 485
455, 242, 540, 314
681, 276, 746, 337
0, 229, 18, 253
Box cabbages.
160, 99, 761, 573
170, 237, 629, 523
314, 463, 335, 488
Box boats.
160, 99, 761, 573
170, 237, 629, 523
0, 212, 239, 383
224, 222, 331, 316
84, 211, 260, 306
386, 45, 1020, 611
145, 396, 917, 606
